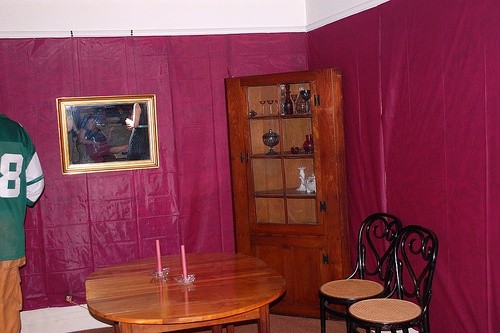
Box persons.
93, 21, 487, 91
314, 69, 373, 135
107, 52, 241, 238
67, 102, 151, 163
0, 113, 45, 333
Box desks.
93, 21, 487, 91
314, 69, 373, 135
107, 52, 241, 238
84, 253, 287, 333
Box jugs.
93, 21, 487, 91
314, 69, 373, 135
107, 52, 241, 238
299, 173, 316, 194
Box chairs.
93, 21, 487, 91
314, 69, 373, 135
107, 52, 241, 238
319, 213, 402, 333
349, 225, 438, 333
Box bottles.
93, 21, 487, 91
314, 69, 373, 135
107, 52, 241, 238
248, 109, 257, 117
295, 82, 310, 114
262, 129, 280, 155
303, 134, 314, 154
284, 84, 293, 115
280, 84, 286, 116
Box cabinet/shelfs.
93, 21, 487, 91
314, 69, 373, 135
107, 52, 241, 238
224, 68, 352, 321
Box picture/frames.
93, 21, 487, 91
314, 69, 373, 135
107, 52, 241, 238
56, 93, 159, 176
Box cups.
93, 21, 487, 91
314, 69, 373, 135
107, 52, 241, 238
291, 146, 299, 154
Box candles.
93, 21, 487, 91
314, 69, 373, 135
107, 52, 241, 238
156, 240, 161, 272
181, 245, 188, 279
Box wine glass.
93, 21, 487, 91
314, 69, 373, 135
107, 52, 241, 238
259, 97, 267, 115
267, 97, 274, 115
290, 93, 298, 114
274, 97, 279, 115
300, 86, 311, 114
